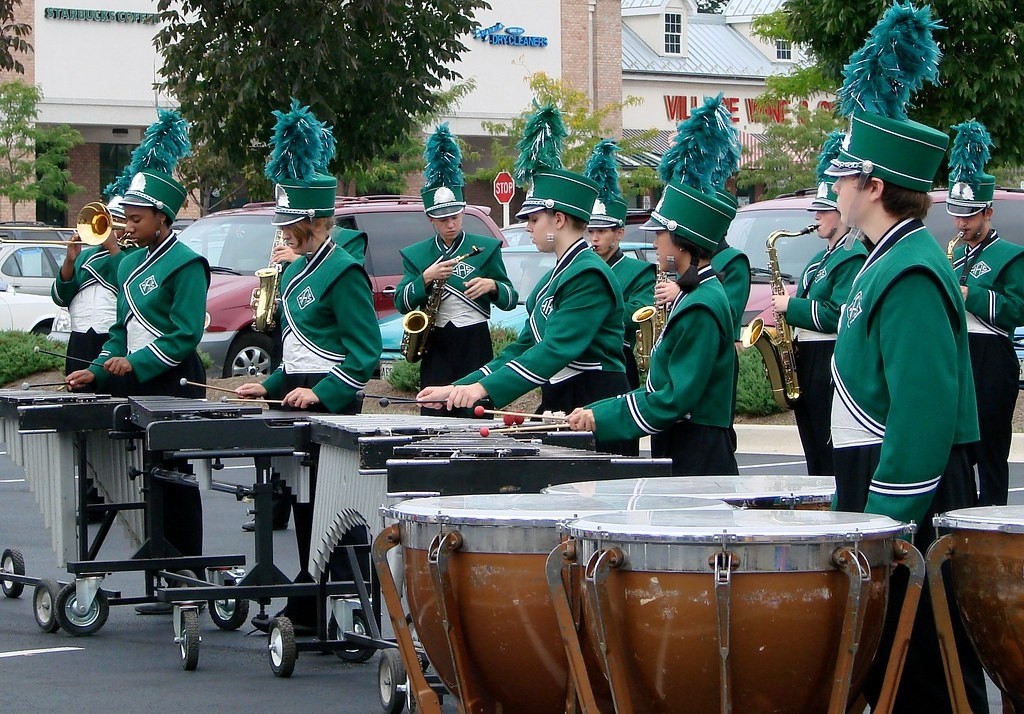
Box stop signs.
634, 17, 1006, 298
493, 173, 515, 205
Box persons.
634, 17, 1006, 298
50, 166, 210, 614
946, 167, 1024, 505
391, 176, 521, 423
830, 108, 992, 714
413, 166, 657, 458
563, 173, 754, 475
236, 173, 383, 632
770, 170, 868, 476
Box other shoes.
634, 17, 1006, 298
134, 601, 205, 615
249, 616, 316, 636
242, 519, 288, 531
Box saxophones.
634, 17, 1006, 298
399, 245, 485, 363
742, 225, 820, 411
249, 228, 287, 333
632, 263, 669, 373
947, 229, 966, 268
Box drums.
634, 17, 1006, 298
541, 475, 836, 510
370, 494, 747, 714
546, 509, 927, 714
927, 505, 1024, 714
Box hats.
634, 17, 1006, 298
515, 94, 601, 223
104, 161, 131, 221
946, 118, 995, 217
703, 126, 739, 207
118, 107, 192, 224
265, 94, 337, 226
584, 137, 628, 229
420, 121, 466, 219
824, 0, 949, 192
638, 91, 738, 254
807, 128, 846, 211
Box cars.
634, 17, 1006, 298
377, 240, 660, 382
0, 217, 233, 340
501, 209, 657, 267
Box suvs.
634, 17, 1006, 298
723, 186, 1024, 352
47, 194, 512, 381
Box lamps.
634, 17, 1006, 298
112, 128, 128, 137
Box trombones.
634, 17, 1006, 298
0, 201, 127, 246
